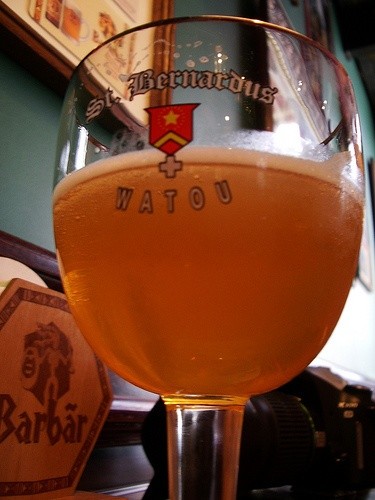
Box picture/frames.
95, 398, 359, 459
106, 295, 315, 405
245, 0, 373, 292
0, 0, 177, 146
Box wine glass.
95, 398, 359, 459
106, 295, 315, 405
49, 11, 365, 500
